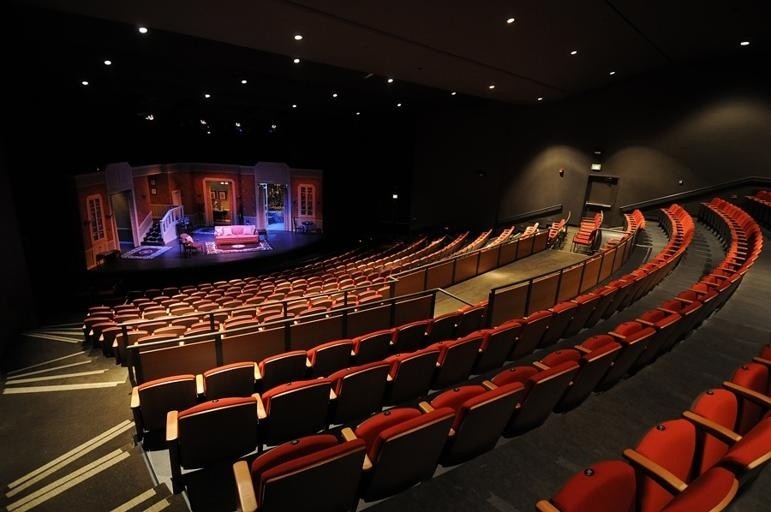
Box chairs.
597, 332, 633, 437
82, 186, 770, 512
